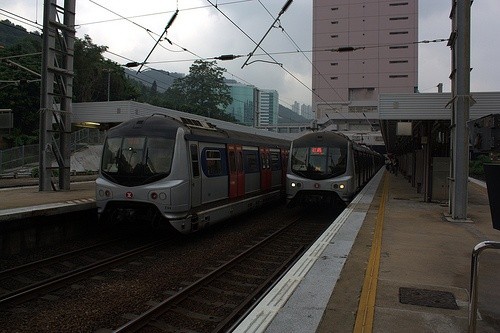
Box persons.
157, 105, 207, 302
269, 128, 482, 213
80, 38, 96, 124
393, 158, 399, 176
384, 157, 395, 174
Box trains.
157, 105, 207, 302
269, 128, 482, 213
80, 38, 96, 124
285, 131, 385, 208
95, 116, 293, 237
